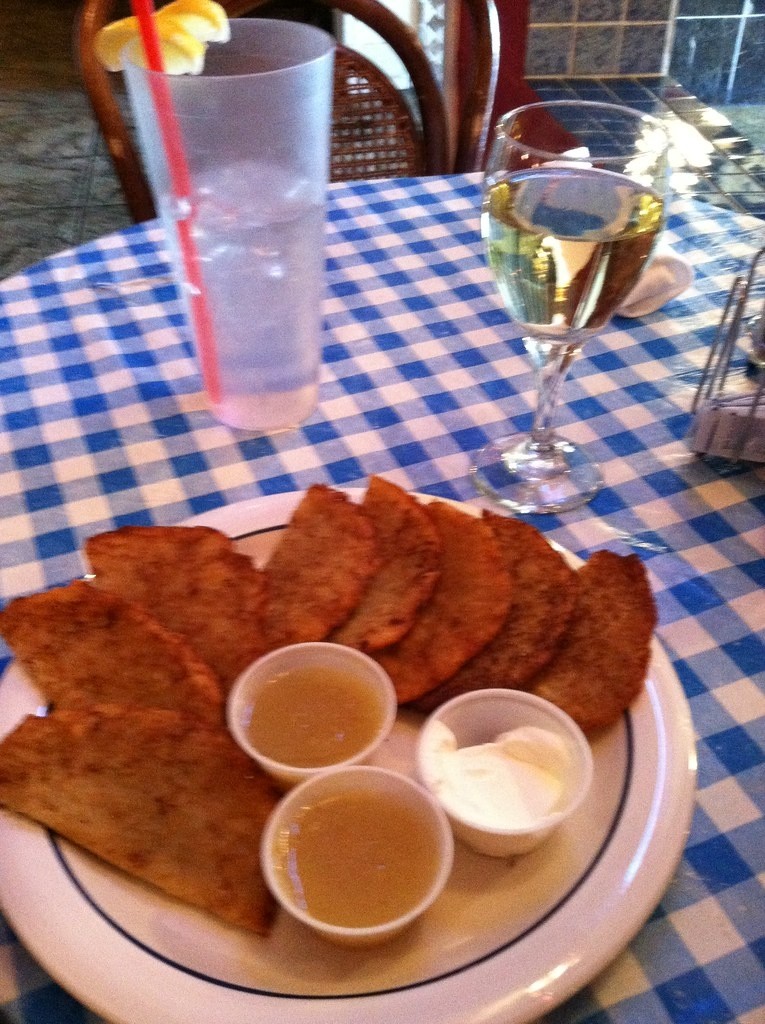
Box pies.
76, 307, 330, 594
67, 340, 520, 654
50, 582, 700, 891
0, 471, 658, 939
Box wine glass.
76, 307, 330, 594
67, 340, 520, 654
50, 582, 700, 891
473, 100, 677, 516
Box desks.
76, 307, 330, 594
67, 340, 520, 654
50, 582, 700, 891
0, 170, 763, 1024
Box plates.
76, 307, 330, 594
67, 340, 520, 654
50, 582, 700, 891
0, 488, 699, 1024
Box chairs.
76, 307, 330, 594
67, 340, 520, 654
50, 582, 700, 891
71, 0, 501, 227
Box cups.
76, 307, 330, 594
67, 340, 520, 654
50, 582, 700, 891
227, 643, 593, 949
121, 20, 335, 431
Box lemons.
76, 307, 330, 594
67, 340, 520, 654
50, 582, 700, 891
93, 0, 230, 78
